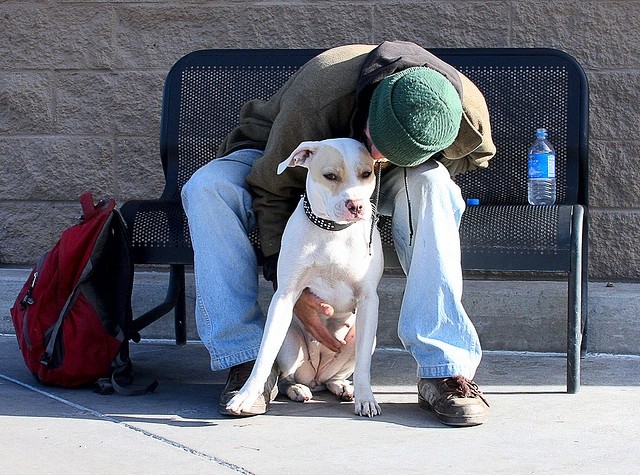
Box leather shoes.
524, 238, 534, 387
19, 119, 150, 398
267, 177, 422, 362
417, 375, 491, 428
217, 360, 281, 417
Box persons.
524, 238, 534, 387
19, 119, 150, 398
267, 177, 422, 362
181, 40, 496, 427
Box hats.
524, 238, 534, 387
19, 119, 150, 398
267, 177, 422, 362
368, 65, 463, 169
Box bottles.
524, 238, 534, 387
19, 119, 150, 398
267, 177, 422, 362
527, 129, 557, 208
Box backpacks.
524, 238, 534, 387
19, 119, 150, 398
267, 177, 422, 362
9, 191, 158, 396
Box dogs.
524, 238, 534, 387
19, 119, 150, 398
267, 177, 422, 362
225, 136, 386, 419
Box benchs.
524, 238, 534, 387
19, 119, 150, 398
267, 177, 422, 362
113, 48, 590, 394
